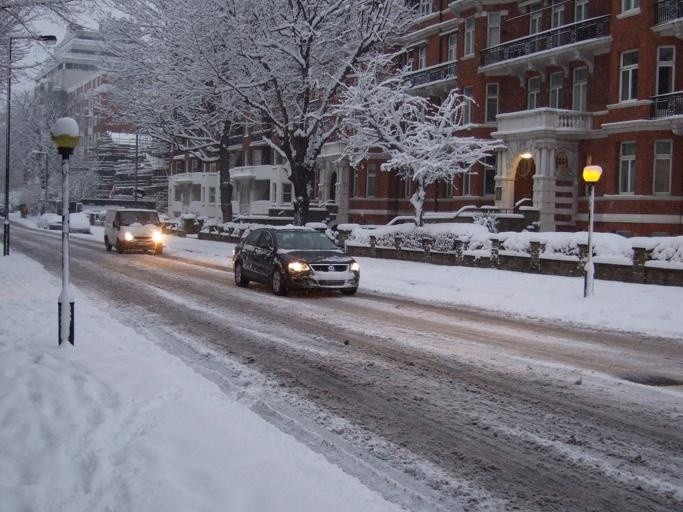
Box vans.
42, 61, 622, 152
102, 206, 165, 255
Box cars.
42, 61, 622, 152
94, 211, 105, 226
232, 224, 361, 298
38, 212, 93, 234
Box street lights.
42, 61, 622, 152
111, 111, 138, 208
580, 163, 602, 297
2, 32, 57, 255
51, 117, 80, 346
30, 148, 48, 200
